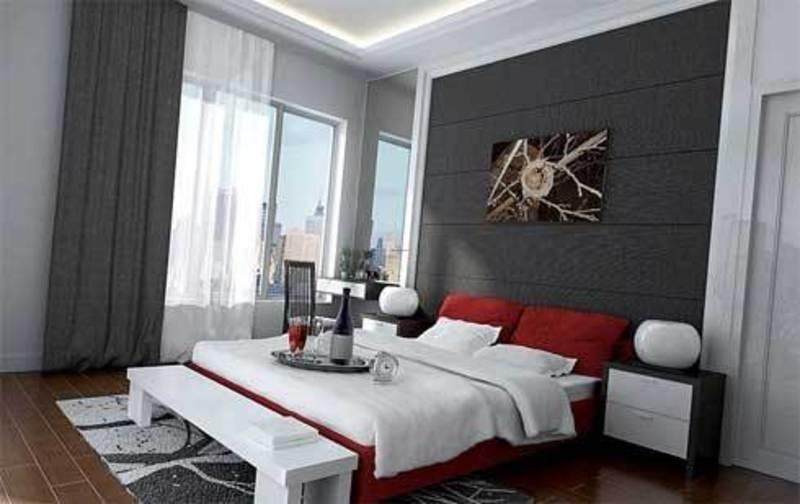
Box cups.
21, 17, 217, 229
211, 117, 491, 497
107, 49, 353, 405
288, 317, 308, 356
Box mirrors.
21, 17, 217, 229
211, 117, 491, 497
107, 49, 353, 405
349, 62, 424, 287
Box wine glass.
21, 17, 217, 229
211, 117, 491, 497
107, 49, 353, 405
306, 319, 324, 355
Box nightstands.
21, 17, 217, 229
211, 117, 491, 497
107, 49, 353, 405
357, 311, 430, 349
592, 359, 728, 481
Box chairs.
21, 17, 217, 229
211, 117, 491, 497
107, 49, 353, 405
281, 258, 337, 335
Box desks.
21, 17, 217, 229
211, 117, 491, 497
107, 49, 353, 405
119, 362, 359, 504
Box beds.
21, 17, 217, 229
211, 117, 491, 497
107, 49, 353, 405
177, 289, 630, 502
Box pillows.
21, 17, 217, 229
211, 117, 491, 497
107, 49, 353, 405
414, 293, 628, 377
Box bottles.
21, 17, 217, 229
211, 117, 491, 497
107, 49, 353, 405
329, 287, 353, 365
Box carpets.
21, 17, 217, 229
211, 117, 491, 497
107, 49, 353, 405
53, 389, 536, 504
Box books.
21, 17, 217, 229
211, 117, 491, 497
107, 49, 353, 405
246, 414, 321, 450
